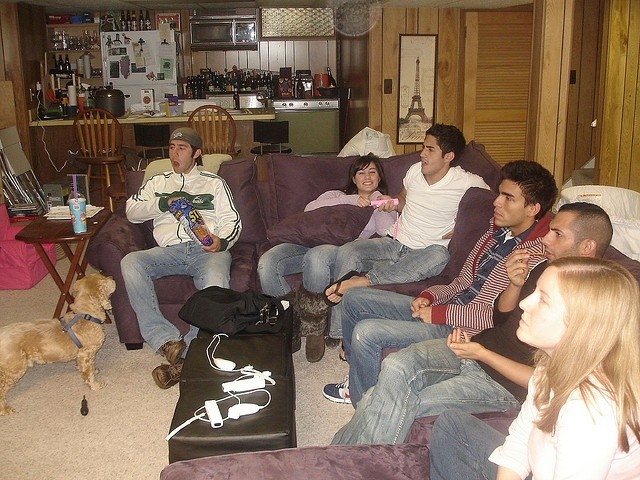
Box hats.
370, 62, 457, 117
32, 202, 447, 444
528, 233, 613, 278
169, 127, 203, 165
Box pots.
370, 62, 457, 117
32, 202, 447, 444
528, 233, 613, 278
89, 85, 131, 118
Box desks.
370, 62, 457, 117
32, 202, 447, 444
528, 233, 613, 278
27, 106, 277, 126
16, 208, 112, 319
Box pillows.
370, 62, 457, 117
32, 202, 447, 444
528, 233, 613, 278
267, 154, 361, 221
123, 159, 269, 245
265, 203, 375, 247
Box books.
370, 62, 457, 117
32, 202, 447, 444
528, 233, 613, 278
45, 204, 96, 217
46, 206, 105, 220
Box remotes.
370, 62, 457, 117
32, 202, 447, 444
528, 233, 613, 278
370, 198, 401, 207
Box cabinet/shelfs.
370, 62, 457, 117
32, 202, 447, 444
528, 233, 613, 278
45, 22, 102, 90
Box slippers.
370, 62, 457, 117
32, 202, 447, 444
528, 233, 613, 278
322, 271, 362, 307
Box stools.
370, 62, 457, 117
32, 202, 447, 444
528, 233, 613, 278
179, 336, 295, 381
167, 379, 296, 463
196, 303, 294, 345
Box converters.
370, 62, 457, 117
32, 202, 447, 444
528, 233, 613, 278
37, 84, 42, 90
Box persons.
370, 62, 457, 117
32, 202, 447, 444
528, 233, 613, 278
331, 199, 613, 445
322, 124, 493, 361
428, 256, 640, 480
258, 154, 398, 361
118, 127, 243, 389
322, 159, 560, 410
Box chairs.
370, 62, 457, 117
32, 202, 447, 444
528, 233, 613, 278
250, 120, 292, 155
134, 124, 170, 171
186, 105, 238, 159
73, 108, 127, 212
1, 126, 44, 205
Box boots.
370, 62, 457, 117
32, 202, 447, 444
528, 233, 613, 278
277, 288, 301, 353
299, 282, 329, 363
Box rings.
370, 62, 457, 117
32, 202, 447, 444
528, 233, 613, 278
420, 301, 428, 307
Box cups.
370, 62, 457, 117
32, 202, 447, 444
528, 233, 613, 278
68, 198, 88, 234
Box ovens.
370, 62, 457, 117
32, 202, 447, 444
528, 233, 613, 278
268, 99, 339, 157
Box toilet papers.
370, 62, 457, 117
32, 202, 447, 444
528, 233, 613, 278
68, 86, 78, 107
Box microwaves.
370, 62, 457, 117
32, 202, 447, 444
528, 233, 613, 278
189, 20, 258, 45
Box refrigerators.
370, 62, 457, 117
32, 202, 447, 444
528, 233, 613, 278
100, 29, 184, 111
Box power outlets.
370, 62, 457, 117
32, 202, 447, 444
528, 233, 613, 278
28, 108, 38, 123
29, 89, 38, 102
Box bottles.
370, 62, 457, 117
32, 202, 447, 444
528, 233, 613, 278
119, 9, 126, 30
145, 9, 152, 30
63, 53, 71, 72
126, 9, 132, 30
186, 71, 274, 97
131, 10, 138, 30
57, 54, 64, 72
138, 9, 144, 30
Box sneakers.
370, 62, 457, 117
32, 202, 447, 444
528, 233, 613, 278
151, 358, 185, 389
161, 340, 186, 364
322, 375, 353, 404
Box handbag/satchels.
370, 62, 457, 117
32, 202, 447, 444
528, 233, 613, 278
178, 286, 285, 337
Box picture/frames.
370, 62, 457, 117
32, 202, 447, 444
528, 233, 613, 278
397, 33, 437, 144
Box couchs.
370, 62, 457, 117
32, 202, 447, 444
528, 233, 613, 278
87, 140, 504, 317
1, 203, 57, 290
158, 187, 640, 480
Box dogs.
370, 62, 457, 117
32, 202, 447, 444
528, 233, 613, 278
1, 273, 117, 417
